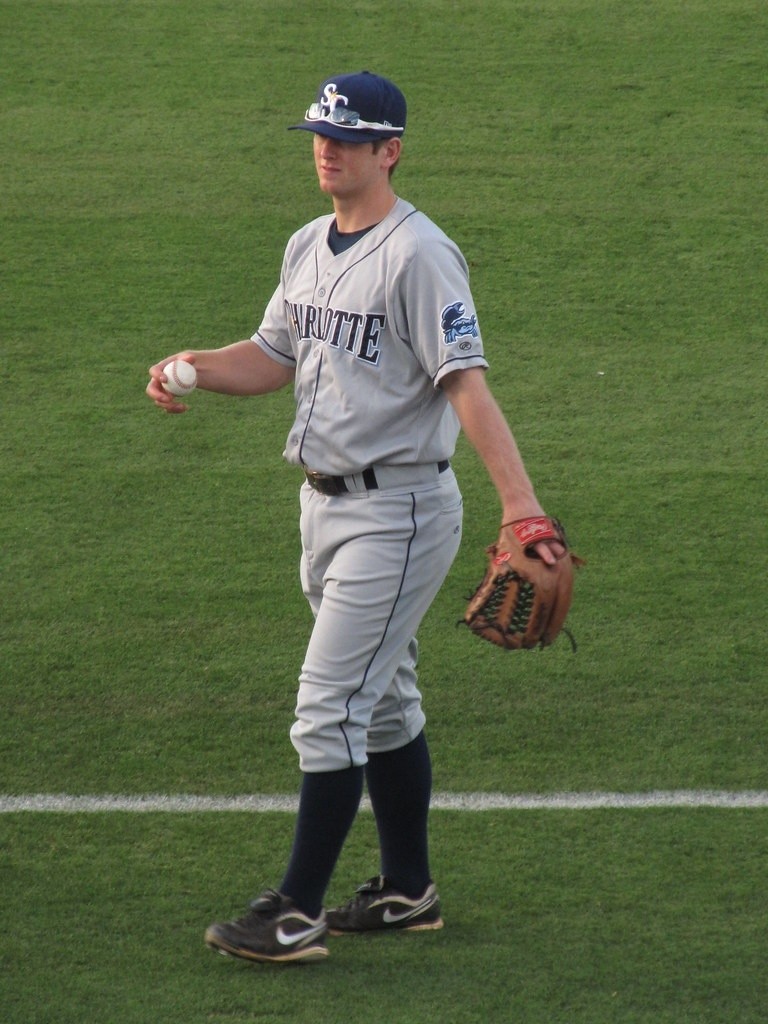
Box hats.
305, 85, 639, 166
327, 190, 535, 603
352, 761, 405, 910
287, 70, 406, 143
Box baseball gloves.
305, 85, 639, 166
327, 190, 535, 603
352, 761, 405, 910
452, 515, 588, 655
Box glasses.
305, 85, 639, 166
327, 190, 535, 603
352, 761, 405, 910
306, 101, 404, 131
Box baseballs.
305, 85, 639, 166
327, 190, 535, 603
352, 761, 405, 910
161, 360, 199, 398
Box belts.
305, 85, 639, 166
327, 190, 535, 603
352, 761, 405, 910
306, 459, 449, 496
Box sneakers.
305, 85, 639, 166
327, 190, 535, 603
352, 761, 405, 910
204, 887, 330, 962
324, 875, 444, 936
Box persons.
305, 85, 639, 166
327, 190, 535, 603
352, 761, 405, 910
146, 70, 576, 964
